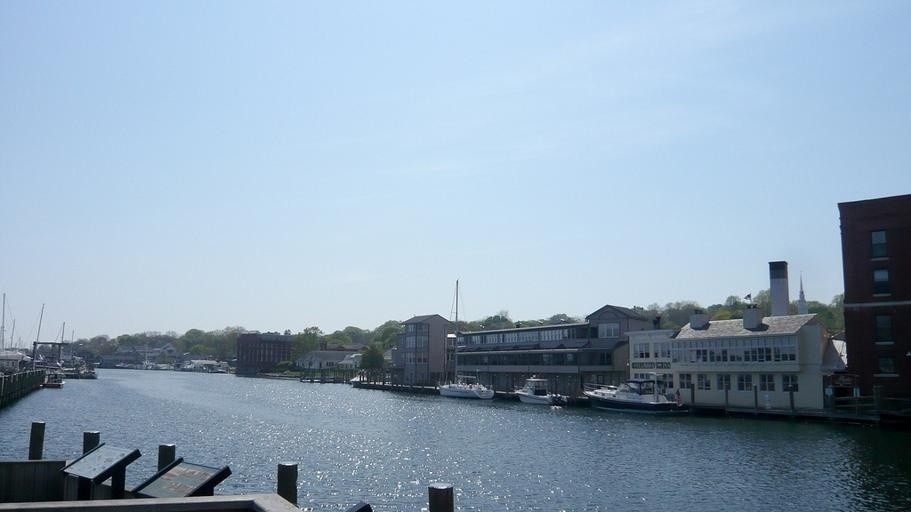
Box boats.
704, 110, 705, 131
43, 374, 65, 388
582, 379, 688, 416
436, 375, 494, 400
515, 379, 570, 406
79, 370, 96, 379
